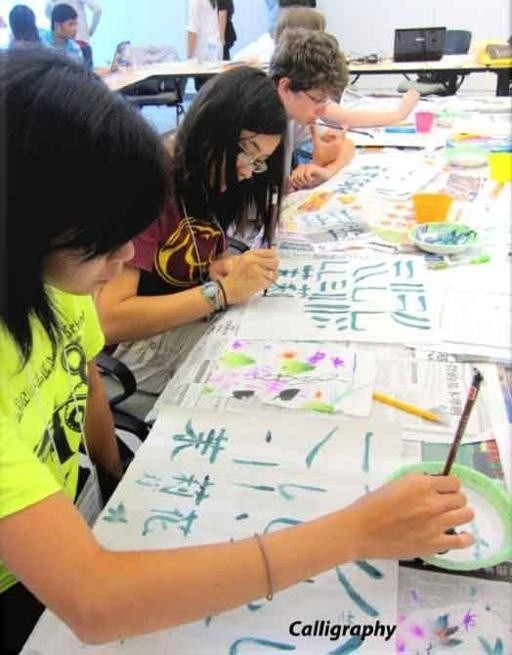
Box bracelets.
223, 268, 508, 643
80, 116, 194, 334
218, 279, 228, 310
254, 531, 274, 601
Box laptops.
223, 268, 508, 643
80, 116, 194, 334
394, 26, 446, 61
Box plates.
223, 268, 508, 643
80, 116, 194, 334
411, 222, 479, 254
386, 461, 512, 572
445, 145, 490, 167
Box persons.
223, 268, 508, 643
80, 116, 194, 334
275, 4, 421, 128
0, 0, 237, 92
94, 65, 287, 347
1, 41, 476, 646
269, 26, 356, 196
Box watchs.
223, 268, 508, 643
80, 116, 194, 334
202, 281, 221, 312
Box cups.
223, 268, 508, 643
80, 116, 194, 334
413, 194, 453, 224
489, 153, 512, 183
416, 113, 433, 134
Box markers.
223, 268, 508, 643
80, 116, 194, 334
386, 128, 415, 133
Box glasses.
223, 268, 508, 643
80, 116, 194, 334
305, 90, 332, 108
239, 143, 268, 174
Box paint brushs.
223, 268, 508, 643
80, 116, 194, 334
307, 122, 373, 138
355, 145, 425, 151
264, 204, 276, 295
442, 368, 483, 476
367, 94, 434, 101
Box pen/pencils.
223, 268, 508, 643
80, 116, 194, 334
372, 392, 439, 423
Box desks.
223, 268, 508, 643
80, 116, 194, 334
20, 96, 512, 651
98, 57, 511, 106
